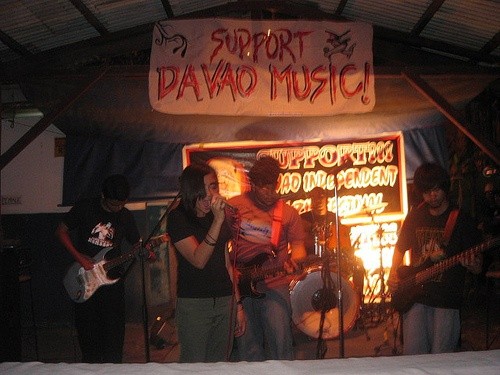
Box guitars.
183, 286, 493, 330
230, 252, 344, 302
63, 234, 170, 303
391, 236, 500, 314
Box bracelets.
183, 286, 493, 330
237, 299, 243, 305
204, 233, 217, 246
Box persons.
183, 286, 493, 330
226, 155, 308, 363
387, 162, 485, 355
56, 172, 157, 363
165, 163, 247, 364
299, 187, 365, 308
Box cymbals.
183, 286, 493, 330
344, 202, 389, 220
371, 230, 395, 235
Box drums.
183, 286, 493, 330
288, 265, 362, 339
317, 223, 352, 254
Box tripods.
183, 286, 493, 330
365, 233, 394, 319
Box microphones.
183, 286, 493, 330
217, 197, 237, 212
330, 159, 353, 175
